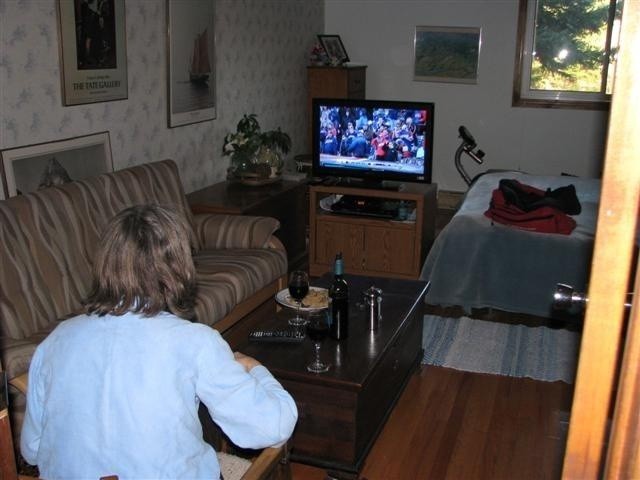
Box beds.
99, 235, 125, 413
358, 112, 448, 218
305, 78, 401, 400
420, 169, 602, 320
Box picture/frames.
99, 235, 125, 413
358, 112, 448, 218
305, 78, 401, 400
219, 113, 261, 153
56, 0, 130, 106
319, 34, 349, 61
166, 0, 217, 128
1, 132, 115, 199
412, 25, 482, 85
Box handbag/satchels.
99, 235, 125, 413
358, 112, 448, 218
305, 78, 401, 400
484, 179, 581, 235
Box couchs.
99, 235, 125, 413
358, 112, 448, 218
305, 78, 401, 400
0, 159, 288, 412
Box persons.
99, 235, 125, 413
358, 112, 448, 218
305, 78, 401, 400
319, 106, 427, 173
19, 203, 300, 479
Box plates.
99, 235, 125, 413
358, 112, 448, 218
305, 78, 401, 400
273, 285, 331, 310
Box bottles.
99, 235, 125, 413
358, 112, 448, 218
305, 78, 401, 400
328, 253, 350, 342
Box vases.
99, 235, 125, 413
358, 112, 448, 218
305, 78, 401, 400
232, 154, 251, 175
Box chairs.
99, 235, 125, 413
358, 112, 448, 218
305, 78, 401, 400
0, 405, 292, 480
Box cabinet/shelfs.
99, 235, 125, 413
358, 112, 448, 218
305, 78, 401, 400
308, 182, 437, 281
308, 65, 366, 152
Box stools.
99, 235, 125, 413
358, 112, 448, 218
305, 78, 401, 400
293, 154, 312, 172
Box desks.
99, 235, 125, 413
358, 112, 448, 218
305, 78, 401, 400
185, 173, 308, 264
220, 273, 430, 479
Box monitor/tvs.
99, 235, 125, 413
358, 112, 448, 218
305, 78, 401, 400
310, 97, 435, 190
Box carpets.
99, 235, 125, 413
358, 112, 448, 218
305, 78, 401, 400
421, 313, 581, 383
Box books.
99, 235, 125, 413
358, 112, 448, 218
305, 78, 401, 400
281, 170, 307, 182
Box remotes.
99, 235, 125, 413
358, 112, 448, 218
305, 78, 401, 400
247, 331, 306, 343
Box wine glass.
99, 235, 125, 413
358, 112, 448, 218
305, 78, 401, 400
305, 310, 330, 373
288, 271, 309, 326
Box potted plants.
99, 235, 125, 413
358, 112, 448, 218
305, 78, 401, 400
310, 43, 327, 65
257, 125, 291, 164
